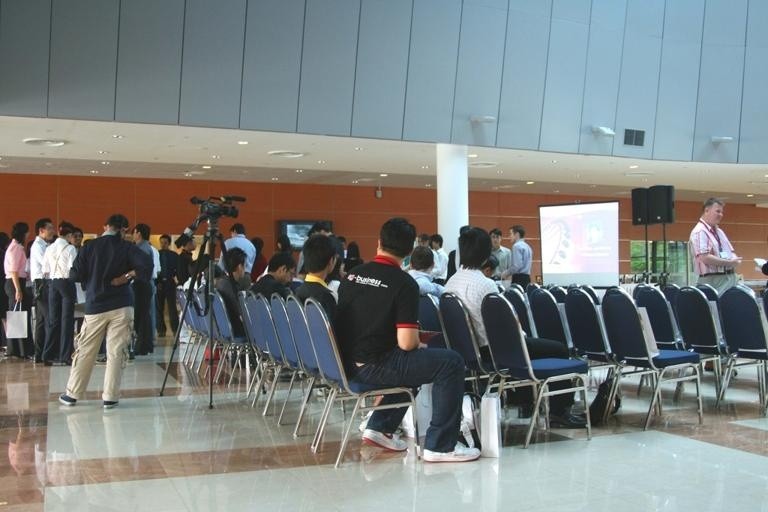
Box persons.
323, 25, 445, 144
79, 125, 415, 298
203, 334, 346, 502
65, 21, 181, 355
332, 216, 482, 465
688, 198, 744, 298
253, 221, 363, 315
762, 261, 767, 319
483, 227, 532, 291
1, 211, 257, 410
442, 227, 594, 428
401, 234, 460, 297
8, 400, 292, 509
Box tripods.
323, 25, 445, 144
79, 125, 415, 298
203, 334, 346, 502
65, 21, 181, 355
159, 227, 266, 409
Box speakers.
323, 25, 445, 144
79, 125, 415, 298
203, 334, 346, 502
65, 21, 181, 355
648, 186, 675, 223
632, 188, 648, 224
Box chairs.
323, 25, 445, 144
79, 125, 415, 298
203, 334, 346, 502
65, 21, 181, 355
173, 278, 767, 463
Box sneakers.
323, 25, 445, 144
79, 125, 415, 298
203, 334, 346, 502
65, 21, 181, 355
103, 402, 120, 409
59, 394, 76, 405
423, 441, 481, 462
362, 429, 409, 452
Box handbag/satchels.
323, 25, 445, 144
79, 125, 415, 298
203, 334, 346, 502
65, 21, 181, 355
6, 301, 27, 339
590, 367, 621, 424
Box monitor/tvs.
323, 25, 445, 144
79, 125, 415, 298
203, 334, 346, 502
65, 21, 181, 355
281, 222, 316, 250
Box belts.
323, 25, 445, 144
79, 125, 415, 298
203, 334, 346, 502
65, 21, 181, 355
698, 271, 735, 278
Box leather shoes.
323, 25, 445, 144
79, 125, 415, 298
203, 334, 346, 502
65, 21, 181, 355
549, 415, 586, 428
518, 404, 542, 418
